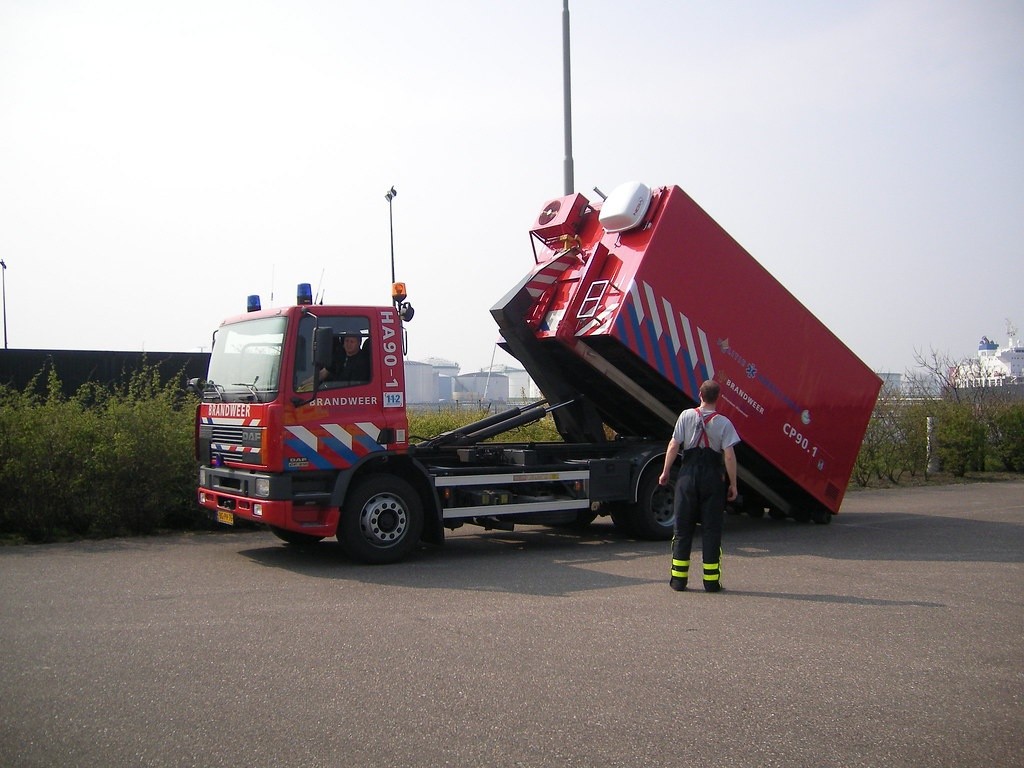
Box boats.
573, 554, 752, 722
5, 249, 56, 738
941, 318, 1024, 403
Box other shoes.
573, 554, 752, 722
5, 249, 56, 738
718, 583, 722, 591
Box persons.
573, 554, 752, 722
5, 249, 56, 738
299, 330, 369, 391
659, 379, 741, 594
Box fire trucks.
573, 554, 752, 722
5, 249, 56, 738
190, 181, 885, 566
